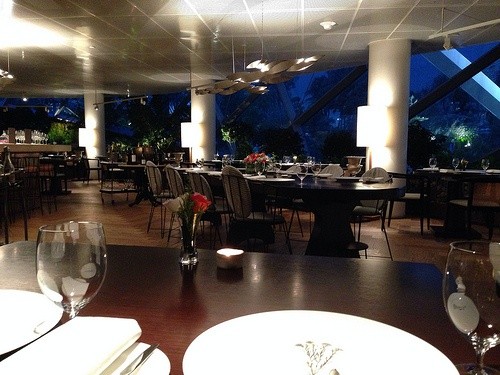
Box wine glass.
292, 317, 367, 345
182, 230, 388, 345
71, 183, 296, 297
35, 219, 109, 320
214, 154, 219, 160
481, 160, 489, 175
429, 158, 438, 173
296, 164, 308, 186
31, 129, 49, 145
442, 238, 500, 375
196, 159, 205, 170
15, 129, 26, 144
254, 154, 291, 178
311, 162, 321, 179
452, 158, 460, 174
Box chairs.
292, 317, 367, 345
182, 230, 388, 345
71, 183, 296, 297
73, 146, 100, 183
145, 160, 500, 254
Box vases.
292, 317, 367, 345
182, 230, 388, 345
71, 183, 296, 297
246, 163, 267, 174
176, 213, 203, 266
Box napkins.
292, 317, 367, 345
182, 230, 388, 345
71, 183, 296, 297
0, 317, 142, 375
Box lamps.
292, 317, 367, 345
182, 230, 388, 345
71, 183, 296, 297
140, 98, 146, 104
94, 105, 99, 111
194, 0, 326, 95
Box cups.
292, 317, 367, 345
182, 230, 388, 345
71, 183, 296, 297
222, 158, 231, 169
216, 248, 244, 269
307, 157, 315, 165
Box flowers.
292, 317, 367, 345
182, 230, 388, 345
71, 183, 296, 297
244, 152, 269, 164
164, 190, 210, 214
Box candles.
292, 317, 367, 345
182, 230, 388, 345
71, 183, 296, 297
215, 247, 245, 271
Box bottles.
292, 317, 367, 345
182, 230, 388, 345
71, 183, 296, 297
126, 147, 146, 165
0, 131, 9, 143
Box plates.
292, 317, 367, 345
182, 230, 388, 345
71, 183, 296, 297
318, 174, 333, 177
181, 309, 462, 374
99, 341, 172, 375
0, 289, 64, 357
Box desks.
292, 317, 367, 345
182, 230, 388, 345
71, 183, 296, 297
0, 164, 500, 375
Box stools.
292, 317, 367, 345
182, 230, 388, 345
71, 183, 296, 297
2, 155, 58, 244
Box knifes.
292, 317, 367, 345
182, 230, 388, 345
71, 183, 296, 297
120, 342, 160, 375
